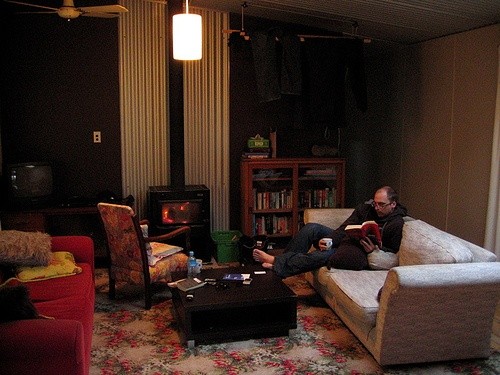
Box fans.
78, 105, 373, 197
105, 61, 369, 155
6, 0, 129, 22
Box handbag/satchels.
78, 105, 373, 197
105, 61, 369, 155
235, 235, 271, 265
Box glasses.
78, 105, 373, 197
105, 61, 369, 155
370, 200, 394, 209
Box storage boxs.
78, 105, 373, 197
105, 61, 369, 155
248, 138, 269, 148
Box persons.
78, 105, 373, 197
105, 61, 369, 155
253, 185, 404, 279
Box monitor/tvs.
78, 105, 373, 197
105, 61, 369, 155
4, 159, 60, 203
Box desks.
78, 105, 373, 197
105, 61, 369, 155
5, 205, 108, 259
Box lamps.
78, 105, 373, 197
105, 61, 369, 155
172, 0, 203, 61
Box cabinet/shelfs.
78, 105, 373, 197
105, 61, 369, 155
145, 184, 213, 261
241, 155, 350, 240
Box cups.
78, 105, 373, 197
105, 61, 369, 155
323, 238, 333, 250
196, 259, 203, 274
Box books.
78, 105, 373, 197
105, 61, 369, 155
298, 213, 305, 232
344, 221, 382, 247
178, 278, 204, 291
253, 188, 293, 210
253, 214, 293, 236
252, 171, 292, 177
304, 170, 337, 177
240, 148, 272, 159
298, 187, 336, 209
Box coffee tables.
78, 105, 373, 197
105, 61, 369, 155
170, 261, 300, 345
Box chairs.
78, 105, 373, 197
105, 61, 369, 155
96, 201, 195, 312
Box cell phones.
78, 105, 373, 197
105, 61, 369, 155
243, 278, 253, 284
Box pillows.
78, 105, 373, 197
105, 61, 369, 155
0, 229, 52, 266
15, 247, 82, 282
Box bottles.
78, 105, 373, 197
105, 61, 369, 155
187, 251, 196, 275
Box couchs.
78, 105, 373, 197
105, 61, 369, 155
0, 225, 96, 375
302, 208, 500, 367
